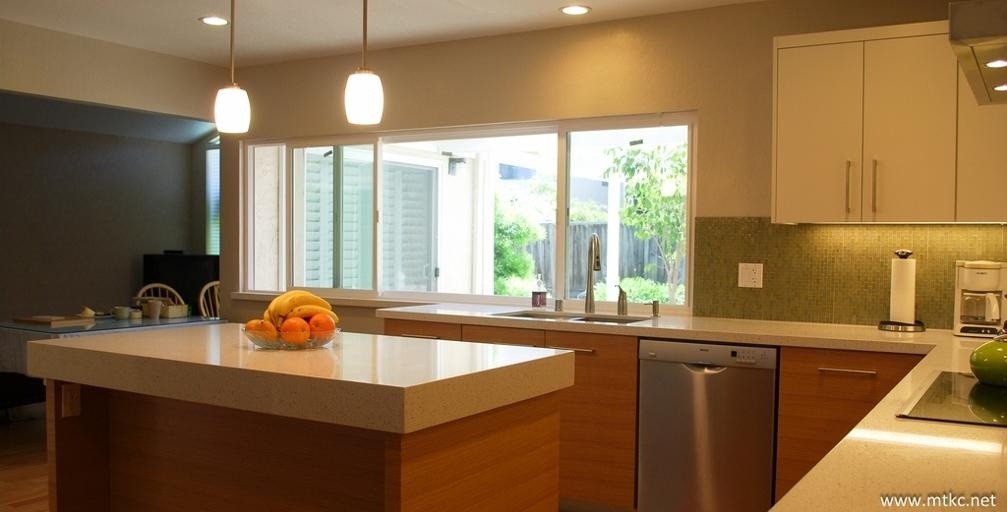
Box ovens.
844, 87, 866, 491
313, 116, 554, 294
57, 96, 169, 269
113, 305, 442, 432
633, 336, 778, 511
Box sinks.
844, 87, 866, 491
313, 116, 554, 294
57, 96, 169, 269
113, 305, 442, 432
490, 310, 580, 320
572, 317, 650, 325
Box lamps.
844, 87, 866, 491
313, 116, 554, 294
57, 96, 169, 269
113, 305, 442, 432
211, 1, 252, 135
342, 1, 386, 129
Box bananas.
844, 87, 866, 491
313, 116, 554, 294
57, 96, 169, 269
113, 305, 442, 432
263, 290, 340, 332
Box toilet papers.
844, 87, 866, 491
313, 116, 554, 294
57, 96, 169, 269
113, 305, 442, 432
890, 258, 916, 325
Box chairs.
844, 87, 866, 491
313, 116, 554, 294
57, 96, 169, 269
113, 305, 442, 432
197, 279, 219, 318
137, 283, 185, 314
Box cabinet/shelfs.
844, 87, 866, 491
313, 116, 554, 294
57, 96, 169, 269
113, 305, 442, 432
771, 345, 924, 506
768, 18, 955, 227
141, 253, 219, 315
955, 62, 1006, 224
543, 329, 638, 509
383, 317, 547, 349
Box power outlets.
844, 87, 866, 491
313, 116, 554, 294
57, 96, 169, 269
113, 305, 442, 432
737, 260, 762, 292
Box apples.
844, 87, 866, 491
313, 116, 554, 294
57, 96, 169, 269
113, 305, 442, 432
280, 317, 310, 344
309, 314, 335, 340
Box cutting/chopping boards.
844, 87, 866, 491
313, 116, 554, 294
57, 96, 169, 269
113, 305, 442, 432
13, 315, 96, 327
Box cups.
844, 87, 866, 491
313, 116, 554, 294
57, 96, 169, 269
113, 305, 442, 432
111, 306, 129, 320
148, 300, 162, 319
128, 310, 142, 319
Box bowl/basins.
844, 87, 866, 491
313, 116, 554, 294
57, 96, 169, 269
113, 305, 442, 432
242, 327, 342, 351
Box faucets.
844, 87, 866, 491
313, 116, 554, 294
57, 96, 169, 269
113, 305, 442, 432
584, 232, 602, 313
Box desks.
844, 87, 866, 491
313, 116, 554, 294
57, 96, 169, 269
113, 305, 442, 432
25, 322, 575, 511
0, 318, 218, 428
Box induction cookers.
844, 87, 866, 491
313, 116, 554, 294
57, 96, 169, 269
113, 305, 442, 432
891, 366, 1007, 427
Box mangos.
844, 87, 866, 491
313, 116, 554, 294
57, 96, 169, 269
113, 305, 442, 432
256, 321, 277, 343
245, 320, 260, 336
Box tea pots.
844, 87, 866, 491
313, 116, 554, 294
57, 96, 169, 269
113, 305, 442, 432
953, 258, 1007, 339
